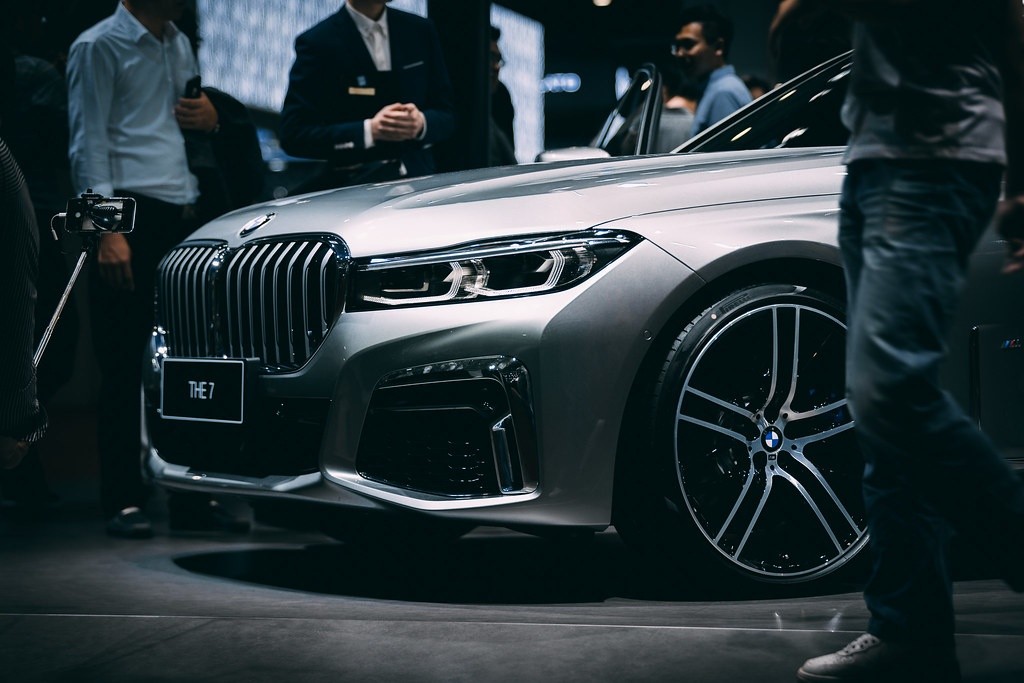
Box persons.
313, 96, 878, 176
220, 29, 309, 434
767, 0, 1024, 683
277, 0, 455, 189
67, 0, 252, 539
655, 6, 769, 153
487, 26, 519, 166
0, 137, 49, 471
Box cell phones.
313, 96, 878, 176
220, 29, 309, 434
185, 75, 202, 100
65, 197, 136, 233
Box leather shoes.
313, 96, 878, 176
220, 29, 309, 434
796, 632, 961, 683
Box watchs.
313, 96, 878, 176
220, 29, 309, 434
206, 123, 220, 137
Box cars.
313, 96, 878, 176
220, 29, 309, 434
136, 47, 1024, 601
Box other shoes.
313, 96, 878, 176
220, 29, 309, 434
108, 507, 154, 538
171, 503, 251, 532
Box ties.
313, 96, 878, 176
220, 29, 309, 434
371, 23, 389, 71
